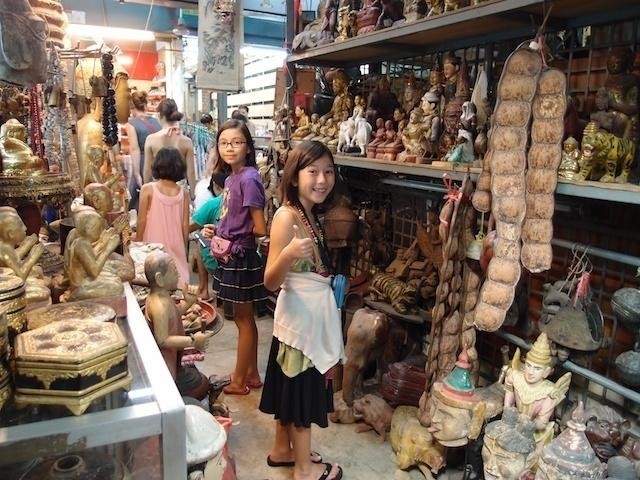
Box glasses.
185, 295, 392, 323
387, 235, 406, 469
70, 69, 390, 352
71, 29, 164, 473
217, 140, 248, 149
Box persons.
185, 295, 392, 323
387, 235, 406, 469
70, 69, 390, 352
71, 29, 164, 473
0, 182, 134, 302
428, 332, 602, 480
590, 46, 640, 140
290, 58, 477, 161
258, 140, 349, 480
121, 91, 254, 300
142, 251, 210, 379
202, 119, 267, 395
83, 146, 121, 190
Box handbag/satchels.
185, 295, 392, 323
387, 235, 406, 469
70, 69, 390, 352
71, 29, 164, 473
211, 236, 232, 259
332, 273, 347, 309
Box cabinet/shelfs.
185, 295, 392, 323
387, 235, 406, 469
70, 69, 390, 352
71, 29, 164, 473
286, 0, 640, 461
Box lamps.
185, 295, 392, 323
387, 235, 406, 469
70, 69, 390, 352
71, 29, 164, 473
65, 23, 154, 41
172, 7, 191, 36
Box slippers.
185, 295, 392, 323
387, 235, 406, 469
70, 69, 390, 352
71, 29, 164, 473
267, 449, 322, 466
222, 372, 263, 395
318, 462, 343, 480
